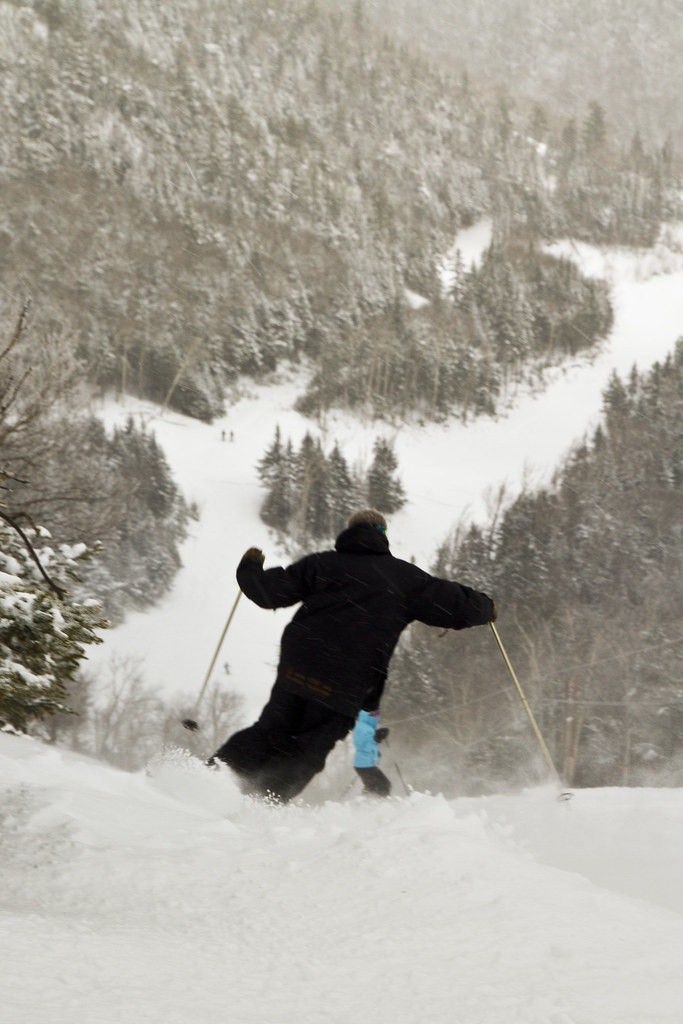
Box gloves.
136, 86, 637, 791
373, 727, 389, 744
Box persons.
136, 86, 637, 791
351, 705, 392, 803
205, 510, 499, 808
